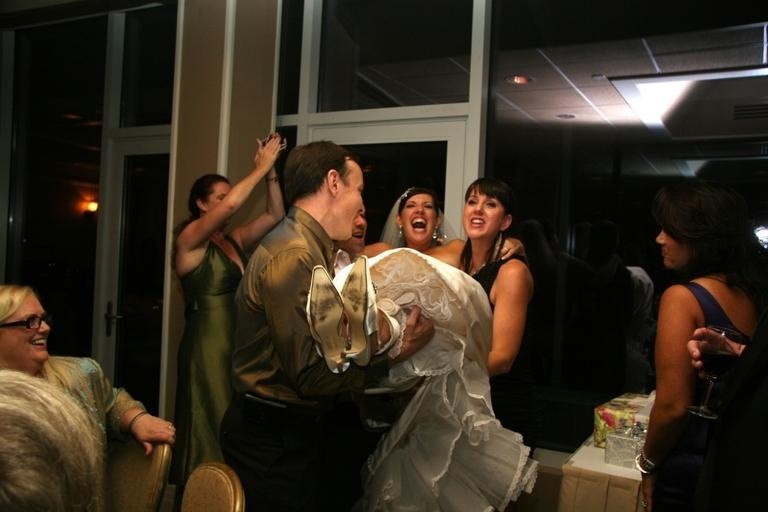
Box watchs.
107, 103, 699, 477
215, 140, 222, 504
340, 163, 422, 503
639, 450, 659, 473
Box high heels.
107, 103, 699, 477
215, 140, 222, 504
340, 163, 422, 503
306, 265, 351, 375
340, 254, 381, 369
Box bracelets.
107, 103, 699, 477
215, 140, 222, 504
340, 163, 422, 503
128, 410, 148, 431
637, 464, 652, 476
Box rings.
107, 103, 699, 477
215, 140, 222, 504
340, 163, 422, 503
168, 423, 172, 428
639, 498, 647, 509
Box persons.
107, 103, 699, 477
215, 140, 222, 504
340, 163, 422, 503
0, 284, 178, 456
687, 304, 768, 511
461, 174, 541, 457
221, 139, 434, 511
169, 131, 287, 488
0, 369, 104, 512
332, 198, 367, 267
633, 179, 768, 512
518, 211, 652, 444
306, 185, 540, 511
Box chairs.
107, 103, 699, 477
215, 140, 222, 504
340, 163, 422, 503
106, 434, 247, 512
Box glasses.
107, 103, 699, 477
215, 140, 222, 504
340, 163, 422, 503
1, 312, 52, 329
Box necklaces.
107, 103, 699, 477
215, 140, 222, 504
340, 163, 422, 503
471, 263, 484, 275
265, 177, 280, 185
211, 233, 225, 245
